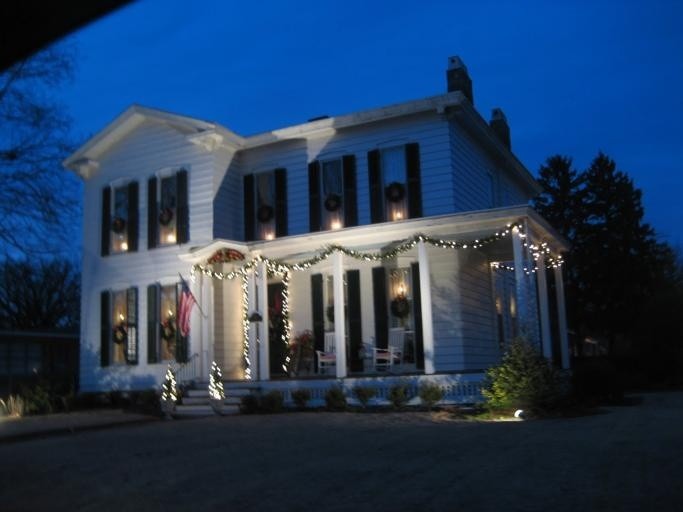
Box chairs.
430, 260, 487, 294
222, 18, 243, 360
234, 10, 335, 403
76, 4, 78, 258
316, 332, 336, 376
371, 328, 405, 372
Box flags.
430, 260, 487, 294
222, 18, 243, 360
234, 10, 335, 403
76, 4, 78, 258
175, 282, 196, 339
